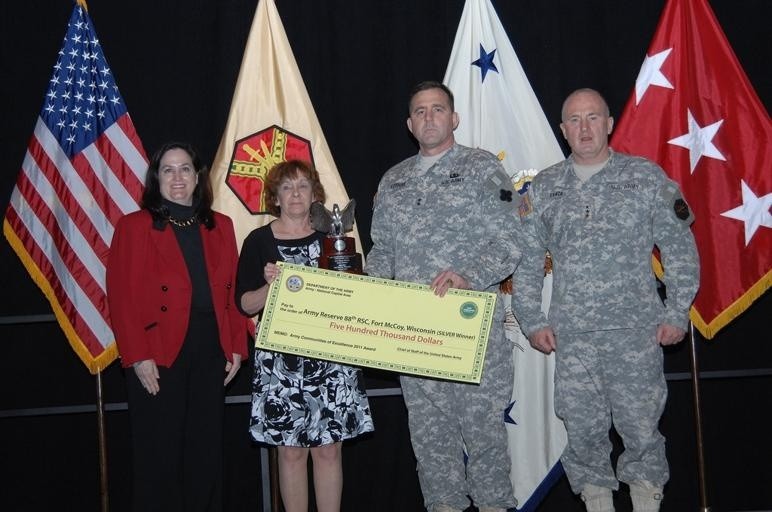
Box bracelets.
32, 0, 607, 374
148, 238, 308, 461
133, 362, 143, 369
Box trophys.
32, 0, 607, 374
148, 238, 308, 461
309, 197, 368, 279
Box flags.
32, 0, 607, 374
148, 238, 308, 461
608, 0, 772, 340
1, 1, 151, 376
440, 1, 570, 510
209, 2, 369, 342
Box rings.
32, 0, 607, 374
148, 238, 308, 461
446, 279, 453, 286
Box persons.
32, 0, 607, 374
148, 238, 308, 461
363, 82, 526, 512
510, 87, 703, 512
235, 160, 365, 512
104, 140, 252, 512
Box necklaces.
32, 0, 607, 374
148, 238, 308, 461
169, 215, 197, 229
